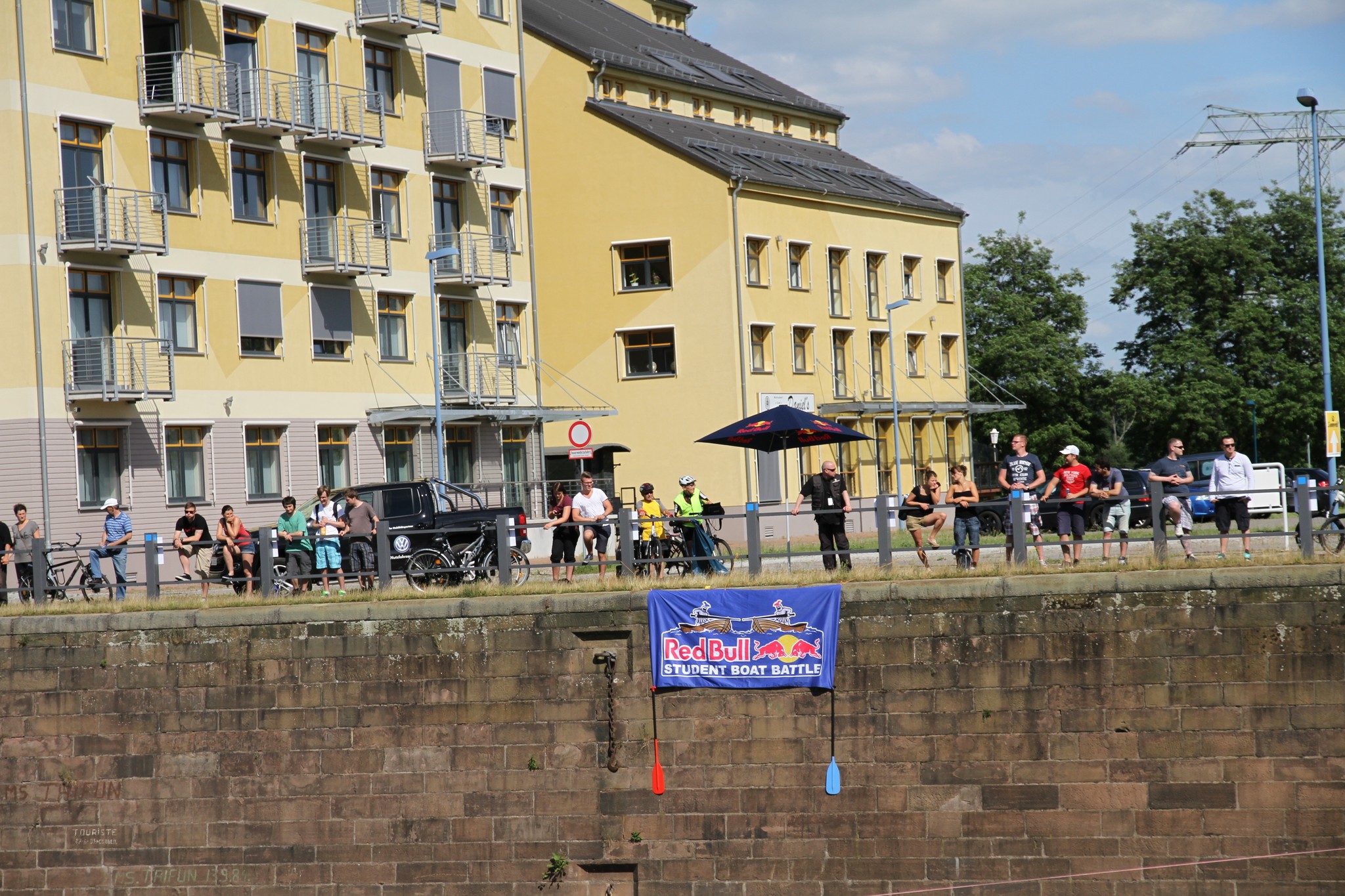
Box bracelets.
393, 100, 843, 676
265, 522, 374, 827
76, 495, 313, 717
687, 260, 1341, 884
174, 539, 178, 544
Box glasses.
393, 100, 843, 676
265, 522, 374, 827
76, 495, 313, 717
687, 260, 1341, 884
643, 491, 653, 495
823, 468, 836, 471
1011, 440, 1022, 444
583, 481, 593, 485
688, 484, 696, 488
184, 510, 195, 514
1174, 445, 1183, 450
1222, 442, 1237, 448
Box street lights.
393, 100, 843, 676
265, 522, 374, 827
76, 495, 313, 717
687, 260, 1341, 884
1246, 400, 1258, 464
424, 244, 462, 512
885, 299, 911, 531
1296, 85, 1337, 534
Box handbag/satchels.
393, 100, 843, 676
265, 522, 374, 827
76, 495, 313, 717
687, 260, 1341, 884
898, 497, 909, 520
951, 545, 974, 573
702, 502, 725, 530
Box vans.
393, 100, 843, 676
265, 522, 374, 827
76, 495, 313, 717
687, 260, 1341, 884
1140, 451, 1225, 490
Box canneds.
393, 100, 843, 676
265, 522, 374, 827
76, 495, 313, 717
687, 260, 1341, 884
1066, 488, 1070, 495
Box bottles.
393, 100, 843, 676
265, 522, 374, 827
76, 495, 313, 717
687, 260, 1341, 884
321, 525, 326, 535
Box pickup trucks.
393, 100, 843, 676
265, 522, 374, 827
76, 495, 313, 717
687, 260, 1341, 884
208, 476, 532, 597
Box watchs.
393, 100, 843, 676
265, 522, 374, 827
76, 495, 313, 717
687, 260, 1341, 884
180, 538, 183, 543
1026, 485, 1030, 490
601, 514, 606, 518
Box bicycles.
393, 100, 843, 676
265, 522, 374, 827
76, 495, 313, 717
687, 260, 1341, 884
403, 521, 531, 593
18, 533, 113, 606
1294, 478, 1345, 559
637, 513, 735, 579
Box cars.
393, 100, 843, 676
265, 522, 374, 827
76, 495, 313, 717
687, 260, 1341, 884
1168, 487, 1216, 525
977, 468, 1152, 537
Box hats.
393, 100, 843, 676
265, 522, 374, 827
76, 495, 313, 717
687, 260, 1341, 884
100, 498, 118, 510
1059, 444, 1079, 456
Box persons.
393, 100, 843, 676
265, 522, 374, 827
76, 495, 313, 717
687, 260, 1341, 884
338, 487, 380, 593
216, 505, 255, 600
1209, 434, 1255, 563
635, 483, 673, 576
1088, 457, 1131, 567
904, 466, 947, 573
277, 496, 313, 598
87, 498, 132, 604
0, 518, 14, 609
1147, 438, 1200, 563
173, 502, 214, 602
310, 485, 347, 598
543, 481, 580, 586
998, 434, 1048, 570
945, 465, 981, 572
673, 475, 713, 572
571, 471, 613, 585
791, 461, 852, 573
1, 504, 40, 606
1040, 444, 1092, 570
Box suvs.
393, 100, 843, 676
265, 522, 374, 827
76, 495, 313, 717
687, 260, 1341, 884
1249, 466, 1295, 519
1278, 467, 1330, 518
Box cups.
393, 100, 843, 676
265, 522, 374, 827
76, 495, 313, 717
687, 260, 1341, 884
1103, 487, 1110, 498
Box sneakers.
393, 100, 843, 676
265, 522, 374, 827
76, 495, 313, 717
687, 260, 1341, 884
582, 553, 594, 563
926, 537, 940, 547
176, 574, 192, 581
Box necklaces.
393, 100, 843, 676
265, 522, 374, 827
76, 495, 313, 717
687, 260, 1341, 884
958, 481, 966, 490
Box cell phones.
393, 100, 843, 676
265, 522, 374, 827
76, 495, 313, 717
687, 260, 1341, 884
555, 512, 560, 514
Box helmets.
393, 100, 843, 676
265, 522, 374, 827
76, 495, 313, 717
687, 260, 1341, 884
639, 483, 654, 493
679, 476, 697, 486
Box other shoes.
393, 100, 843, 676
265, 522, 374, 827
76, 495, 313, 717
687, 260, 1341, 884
222, 572, 235, 579
1185, 553, 1200, 564
1099, 556, 1109, 566
1245, 548, 1253, 563
1118, 556, 1128, 566
87, 578, 103, 585
1039, 559, 1048, 569
1057, 559, 1080, 569
1216, 552, 1228, 562
337, 589, 347, 597
321, 590, 331, 598
1174, 521, 1184, 538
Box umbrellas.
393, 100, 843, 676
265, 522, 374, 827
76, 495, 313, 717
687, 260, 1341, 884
693, 404, 876, 573
